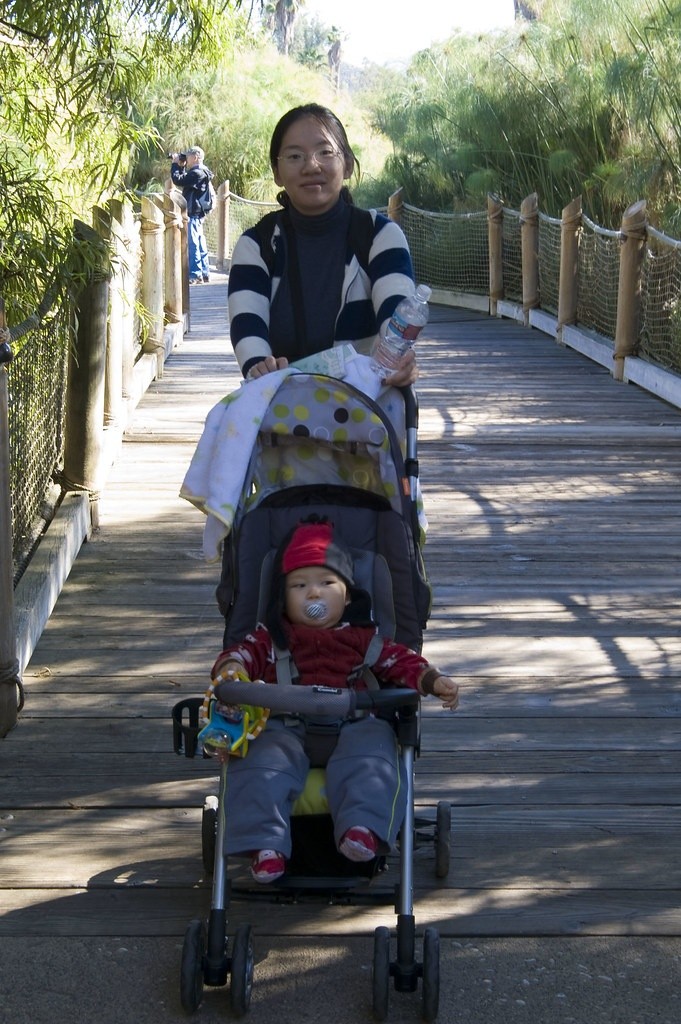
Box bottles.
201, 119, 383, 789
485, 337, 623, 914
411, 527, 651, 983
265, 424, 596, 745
370, 284, 432, 380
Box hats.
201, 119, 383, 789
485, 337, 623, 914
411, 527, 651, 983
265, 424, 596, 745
181, 146, 205, 156
278, 520, 356, 582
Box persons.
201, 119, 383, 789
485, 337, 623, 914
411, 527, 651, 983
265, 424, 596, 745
171, 146, 215, 285
228, 103, 420, 387
211, 514, 460, 883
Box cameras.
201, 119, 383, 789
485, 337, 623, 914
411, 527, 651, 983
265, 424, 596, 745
166, 153, 186, 162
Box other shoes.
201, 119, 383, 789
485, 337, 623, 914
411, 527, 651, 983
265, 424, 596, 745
340, 826, 380, 862
204, 274, 210, 282
251, 851, 288, 884
190, 277, 205, 284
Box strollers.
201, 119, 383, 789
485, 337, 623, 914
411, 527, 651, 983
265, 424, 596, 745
170, 370, 453, 1024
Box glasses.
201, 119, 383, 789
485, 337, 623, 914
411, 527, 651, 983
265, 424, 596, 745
275, 145, 347, 166
